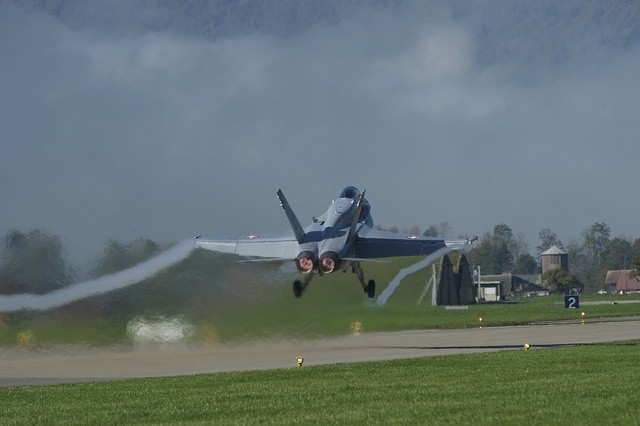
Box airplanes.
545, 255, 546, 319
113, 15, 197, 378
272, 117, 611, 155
198, 187, 480, 298
